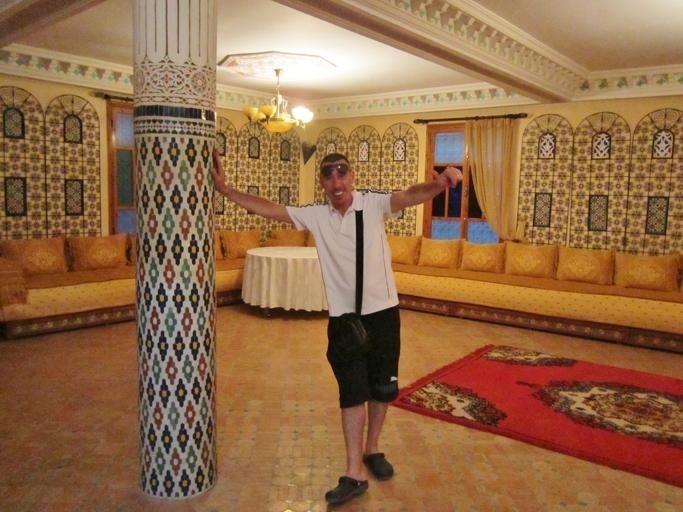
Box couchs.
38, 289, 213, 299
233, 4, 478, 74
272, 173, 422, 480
0, 223, 683, 351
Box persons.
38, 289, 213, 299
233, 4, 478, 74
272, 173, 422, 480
209, 147, 464, 501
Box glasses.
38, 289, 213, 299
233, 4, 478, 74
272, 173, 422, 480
321, 163, 348, 176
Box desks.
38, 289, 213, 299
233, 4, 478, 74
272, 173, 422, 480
238, 246, 327, 315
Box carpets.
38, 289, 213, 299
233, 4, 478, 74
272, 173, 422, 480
377, 342, 683, 493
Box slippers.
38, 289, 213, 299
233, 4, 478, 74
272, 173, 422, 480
363, 450, 393, 481
325, 476, 368, 503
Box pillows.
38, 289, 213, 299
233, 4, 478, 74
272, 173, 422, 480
383, 233, 421, 269
555, 244, 615, 286
455, 238, 505, 275
504, 239, 558, 279
416, 235, 465, 270
219, 227, 262, 259
613, 249, 683, 292
212, 230, 222, 261
126, 233, 138, 267
66, 233, 128, 272
270, 229, 307, 247
5, 236, 67, 275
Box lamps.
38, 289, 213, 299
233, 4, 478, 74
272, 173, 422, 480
240, 65, 315, 136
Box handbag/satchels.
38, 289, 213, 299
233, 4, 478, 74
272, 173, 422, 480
328, 312, 373, 362
